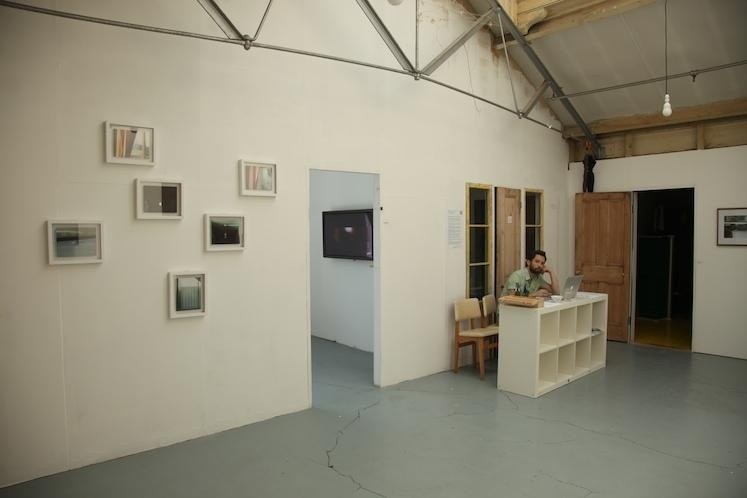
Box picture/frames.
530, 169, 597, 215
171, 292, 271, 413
135, 178, 184, 220
717, 207, 747, 246
46, 218, 104, 266
105, 120, 158, 167
239, 159, 279, 197
168, 269, 209, 318
203, 213, 247, 252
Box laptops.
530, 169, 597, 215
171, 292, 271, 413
540, 275, 584, 300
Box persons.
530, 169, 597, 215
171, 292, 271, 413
500, 250, 560, 303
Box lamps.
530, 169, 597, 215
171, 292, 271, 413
662, 0, 673, 117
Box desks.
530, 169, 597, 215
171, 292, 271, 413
497, 291, 608, 399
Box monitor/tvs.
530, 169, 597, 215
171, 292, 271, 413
323, 209, 372, 259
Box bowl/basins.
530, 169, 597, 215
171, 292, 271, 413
551, 294, 563, 302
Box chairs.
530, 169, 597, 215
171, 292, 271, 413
481, 293, 499, 362
453, 294, 499, 380
